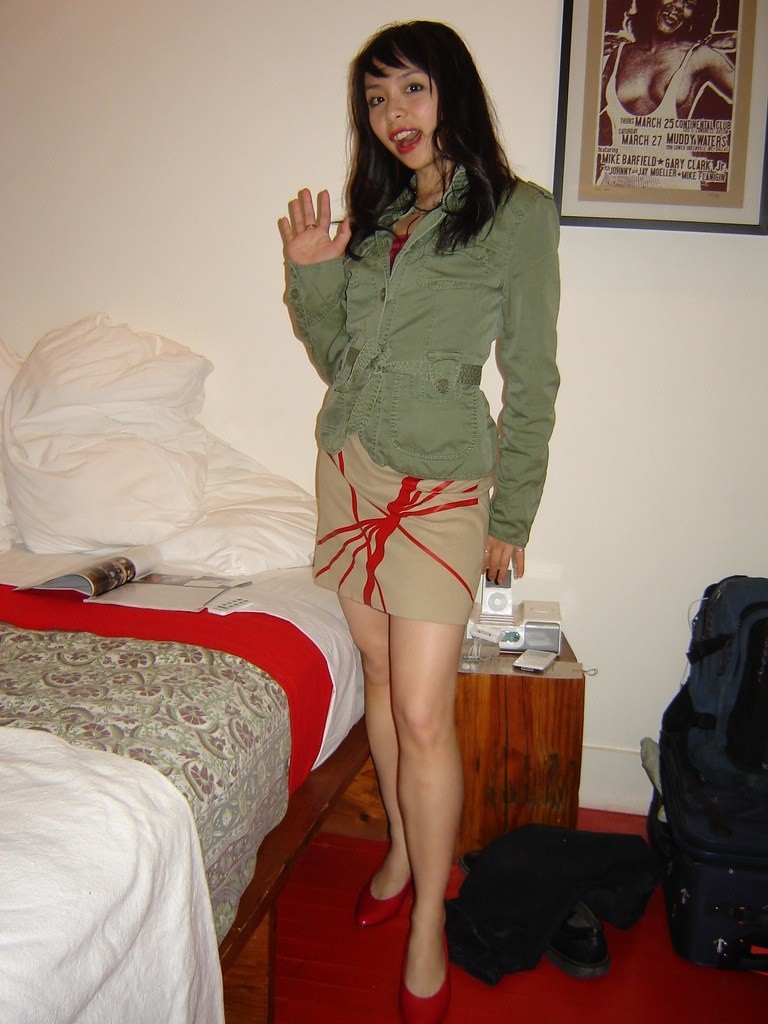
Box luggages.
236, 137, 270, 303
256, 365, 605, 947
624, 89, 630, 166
666, 850, 767, 974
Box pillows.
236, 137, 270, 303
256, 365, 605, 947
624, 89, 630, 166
1, 312, 321, 578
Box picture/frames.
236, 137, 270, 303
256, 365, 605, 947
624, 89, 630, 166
554, 0, 768, 235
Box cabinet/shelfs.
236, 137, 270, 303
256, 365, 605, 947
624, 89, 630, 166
452, 630, 586, 866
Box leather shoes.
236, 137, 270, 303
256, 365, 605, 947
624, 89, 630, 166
356, 869, 415, 927
460, 850, 478, 872
548, 898, 610, 979
399, 925, 449, 1024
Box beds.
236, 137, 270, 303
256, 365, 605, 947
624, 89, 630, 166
0, 567, 368, 1024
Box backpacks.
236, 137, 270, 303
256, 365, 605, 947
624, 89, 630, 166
649, 573, 768, 860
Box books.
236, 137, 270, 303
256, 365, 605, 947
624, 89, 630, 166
14, 548, 253, 613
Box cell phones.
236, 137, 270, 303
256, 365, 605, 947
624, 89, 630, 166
514, 648, 556, 674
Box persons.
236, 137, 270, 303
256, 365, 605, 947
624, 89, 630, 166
279, 22, 561, 1024
598, 0, 735, 198
